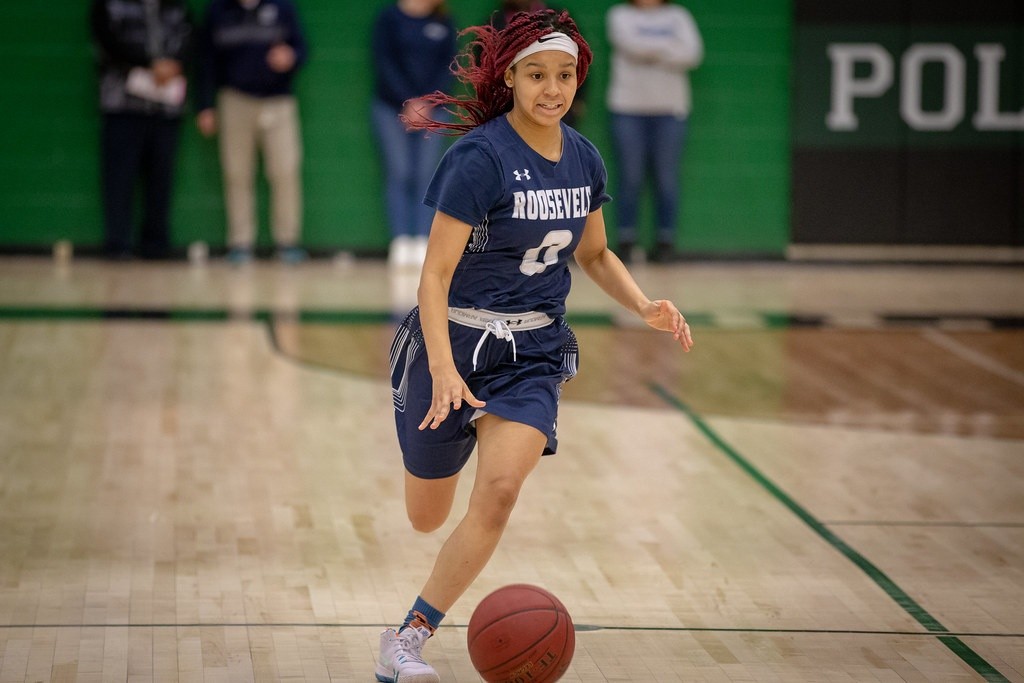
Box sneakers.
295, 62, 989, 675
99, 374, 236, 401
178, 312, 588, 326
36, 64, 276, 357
374, 626, 439, 682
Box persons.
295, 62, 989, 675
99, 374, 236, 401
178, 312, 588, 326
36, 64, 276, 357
370, 0, 458, 273
602, 1, 706, 265
87, 0, 204, 260
373, 9, 694, 683
188, 0, 314, 264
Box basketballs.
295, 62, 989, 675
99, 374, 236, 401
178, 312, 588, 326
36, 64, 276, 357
467, 583, 576, 683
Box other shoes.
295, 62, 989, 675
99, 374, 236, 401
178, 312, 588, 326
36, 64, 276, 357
615, 239, 636, 264
270, 247, 308, 264
648, 241, 673, 265
224, 248, 255, 262
388, 234, 429, 269
